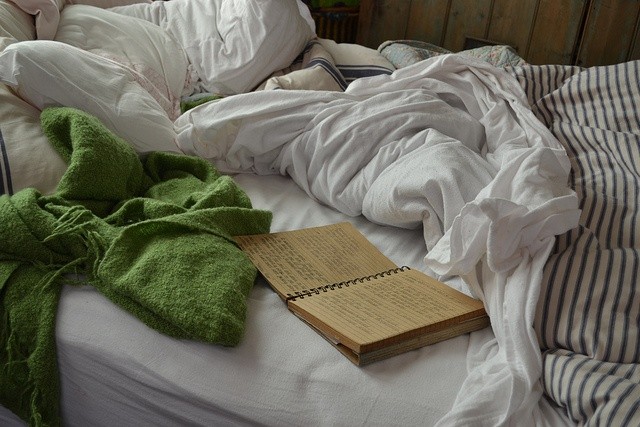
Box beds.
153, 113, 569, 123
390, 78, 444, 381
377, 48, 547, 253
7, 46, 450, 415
1, 0, 640, 427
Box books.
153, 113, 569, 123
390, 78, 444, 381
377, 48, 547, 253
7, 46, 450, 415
231, 221, 490, 368
